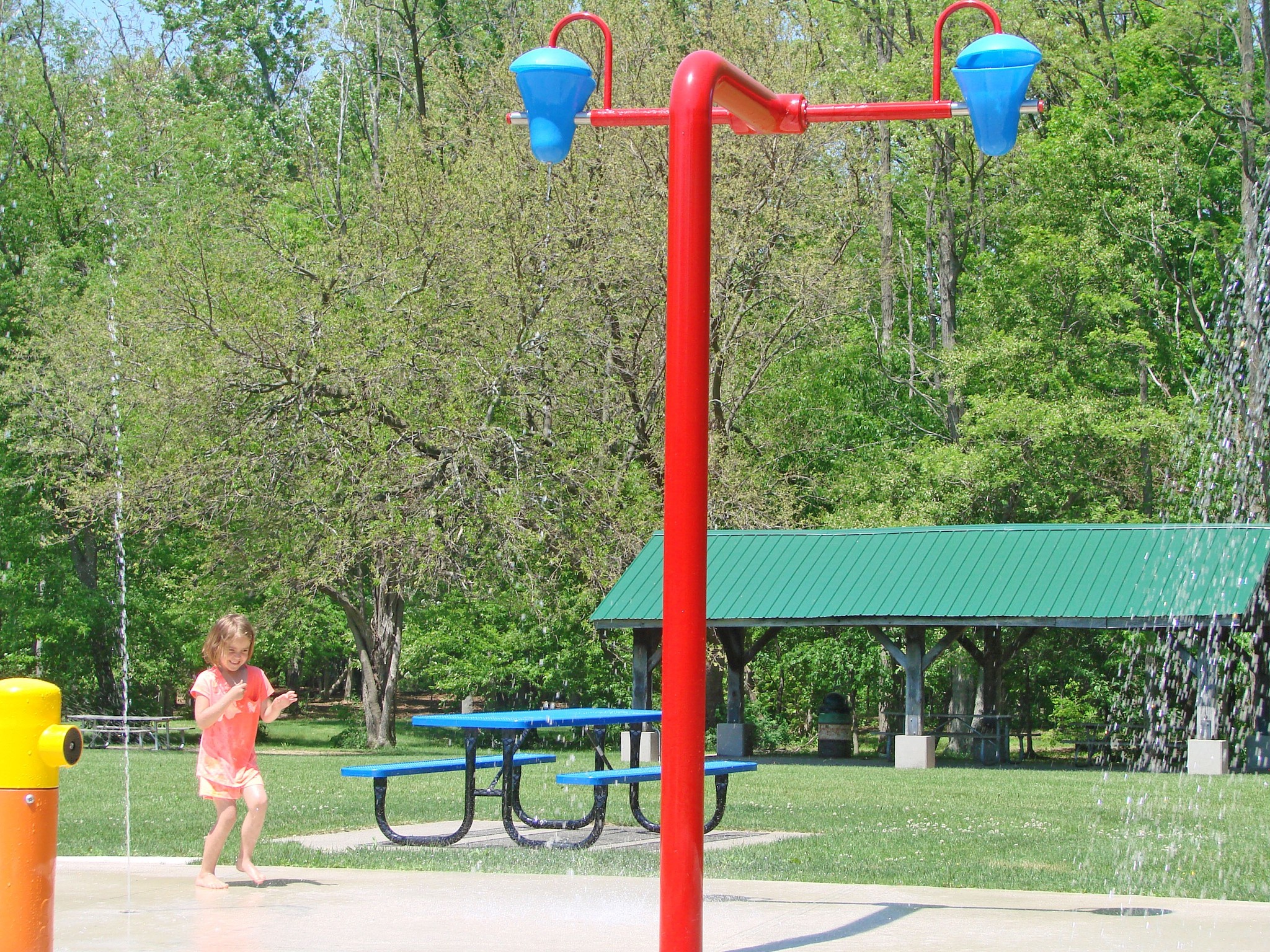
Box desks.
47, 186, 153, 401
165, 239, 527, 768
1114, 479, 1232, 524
1068, 722, 1196, 763
412, 710, 662, 850
880, 711, 1014, 762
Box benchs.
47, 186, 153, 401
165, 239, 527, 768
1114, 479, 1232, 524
1059, 740, 1192, 761
556, 760, 758, 850
1008, 733, 1040, 761
1075, 741, 1187, 769
341, 753, 556, 847
870, 731, 1005, 763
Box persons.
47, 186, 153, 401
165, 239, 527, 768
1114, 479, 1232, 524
190, 613, 297, 889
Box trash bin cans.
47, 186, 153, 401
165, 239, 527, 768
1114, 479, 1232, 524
973, 713, 1006, 765
817, 692, 853, 757
